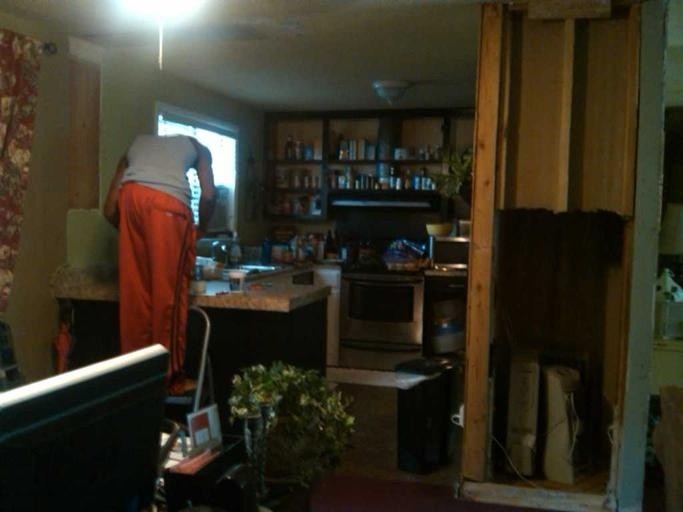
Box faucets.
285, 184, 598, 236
209, 238, 239, 269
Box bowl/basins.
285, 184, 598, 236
426, 223, 451, 237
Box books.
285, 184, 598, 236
327, 135, 438, 191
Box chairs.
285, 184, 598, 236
165, 304, 218, 413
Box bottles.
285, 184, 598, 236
294, 232, 308, 267
262, 232, 272, 266
317, 230, 348, 259
271, 132, 441, 191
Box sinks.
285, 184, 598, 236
222, 263, 284, 277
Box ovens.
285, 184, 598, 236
340, 271, 425, 345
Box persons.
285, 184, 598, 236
102, 133, 218, 397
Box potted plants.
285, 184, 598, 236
226, 359, 361, 512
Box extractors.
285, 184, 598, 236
329, 193, 441, 213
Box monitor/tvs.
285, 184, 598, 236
0, 343, 170, 511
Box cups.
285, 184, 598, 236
229, 272, 246, 293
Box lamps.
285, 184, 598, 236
372, 79, 409, 105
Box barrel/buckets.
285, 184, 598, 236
424, 291, 466, 356
653, 270, 683, 340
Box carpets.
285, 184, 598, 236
309, 469, 574, 512
